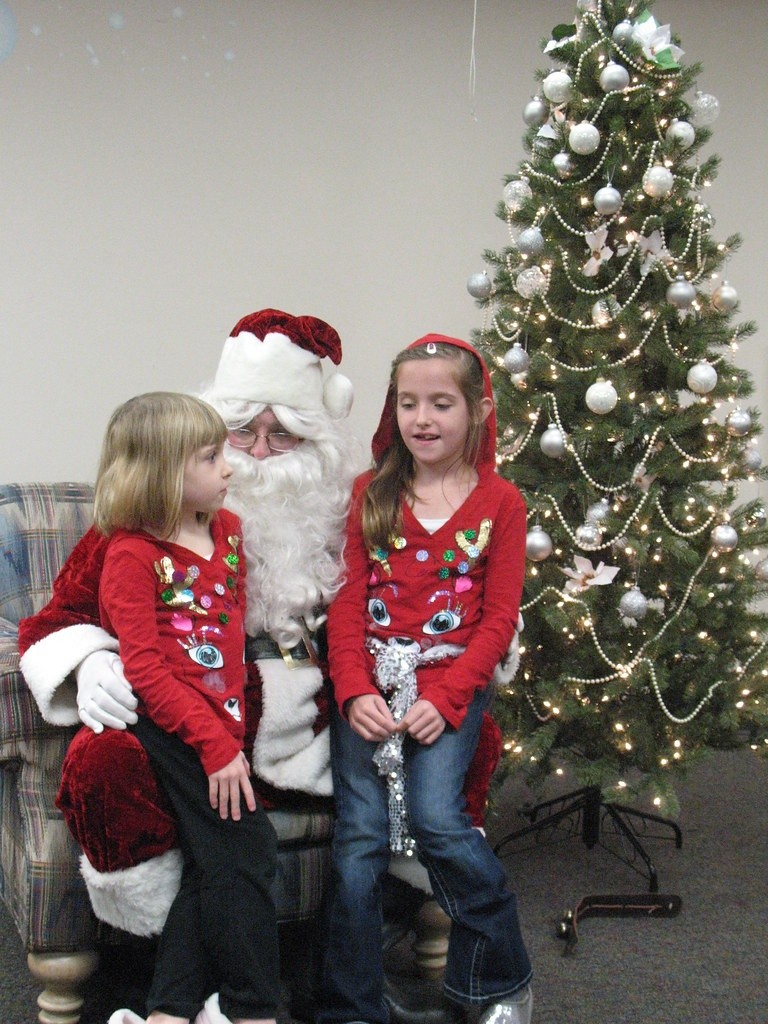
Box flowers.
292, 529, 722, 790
559, 554, 621, 596
581, 228, 613, 278
635, 232, 668, 275
630, 464, 658, 493
630, 14, 685, 68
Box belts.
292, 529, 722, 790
245, 628, 329, 669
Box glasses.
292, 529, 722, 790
226, 427, 301, 452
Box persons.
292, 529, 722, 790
91, 392, 288, 1023
318, 330, 535, 1024
14, 307, 525, 1023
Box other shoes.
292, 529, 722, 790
108, 1008, 146, 1024
193, 991, 236, 1024
478, 981, 533, 1024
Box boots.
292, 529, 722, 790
372, 827, 490, 1024
80, 846, 187, 1024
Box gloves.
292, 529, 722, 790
500, 611, 525, 672
74, 650, 138, 734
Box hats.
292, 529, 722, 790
214, 308, 353, 421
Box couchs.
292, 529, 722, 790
0, 484, 523, 1024
